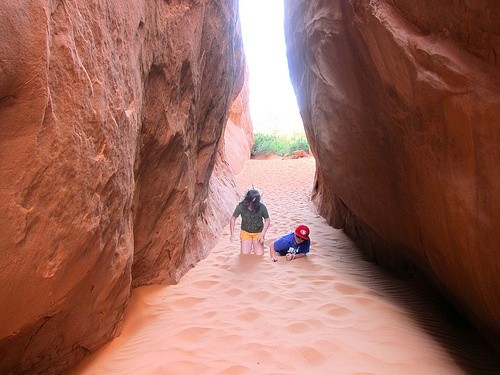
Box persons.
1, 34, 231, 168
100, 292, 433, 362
229, 190, 271, 255
271, 225, 310, 262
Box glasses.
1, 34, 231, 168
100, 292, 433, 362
296, 235, 305, 240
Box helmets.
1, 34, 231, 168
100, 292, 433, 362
295, 225, 310, 240
